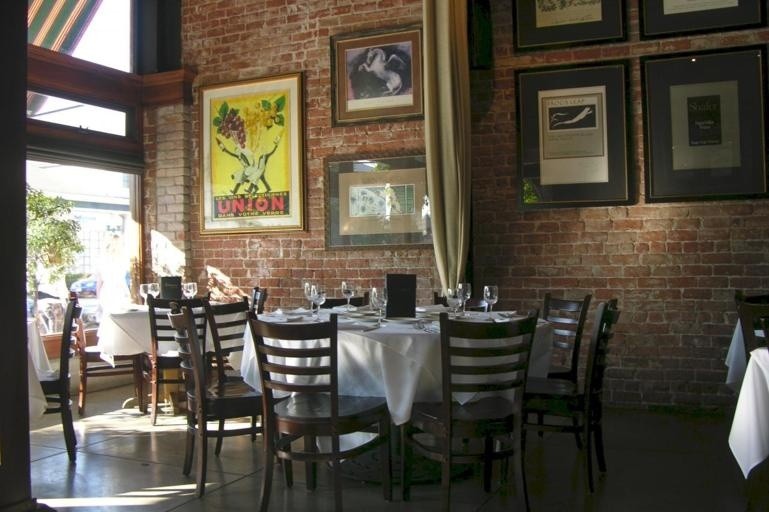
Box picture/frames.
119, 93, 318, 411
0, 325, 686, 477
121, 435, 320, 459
511, 0, 629, 53
637, 0, 767, 42
199, 73, 309, 236
329, 24, 424, 128
638, 43, 769, 204
325, 150, 436, 249
513, 58, 636, 210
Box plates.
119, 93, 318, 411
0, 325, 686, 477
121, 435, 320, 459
257, 304, 520, 331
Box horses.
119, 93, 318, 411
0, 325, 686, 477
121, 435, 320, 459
358, 48, 404, 96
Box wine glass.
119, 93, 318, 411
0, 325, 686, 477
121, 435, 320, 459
139, 282, 159, 309
340, 282, 358, 311
183, 283, 197, 299
304, 283, 325, 323
447, 283, 498, 321
372, 288, 388, 328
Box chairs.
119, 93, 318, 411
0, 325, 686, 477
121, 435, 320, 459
399, 307, 539, 511
481, 292, 621, 495
727, 291, 769, 365
39, 299, 82, 462
246, 311, 394, 512
